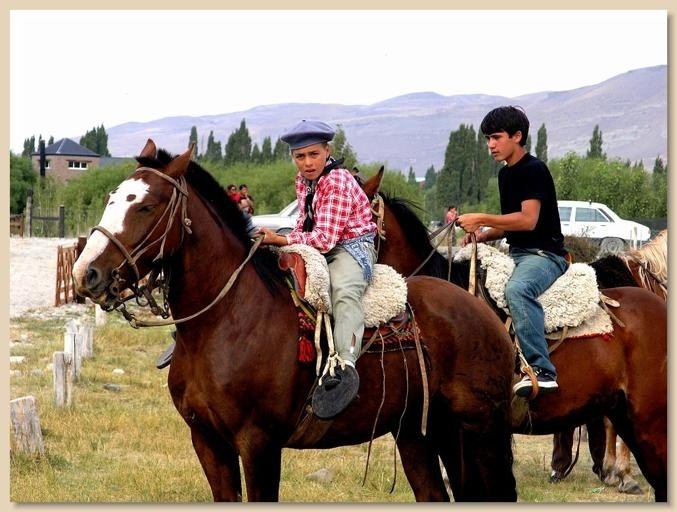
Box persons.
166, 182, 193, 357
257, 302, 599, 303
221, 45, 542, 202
445, 205, 457, 247
237, 184, 255, 215
453, 104, 571, 398
249, 119, 379, 403
224, 184, 241, 204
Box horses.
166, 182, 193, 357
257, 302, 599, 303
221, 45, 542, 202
348, 162, 666, 504
600, 225, 667, 494
71, 137, 519, 501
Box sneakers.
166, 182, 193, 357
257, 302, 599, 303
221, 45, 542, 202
511, 367, 559, 397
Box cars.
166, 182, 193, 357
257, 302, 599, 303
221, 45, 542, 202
555, 199, 650, 255
247, 198, 300, 237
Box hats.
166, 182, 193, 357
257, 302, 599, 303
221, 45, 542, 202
279, 119, 335, 150
